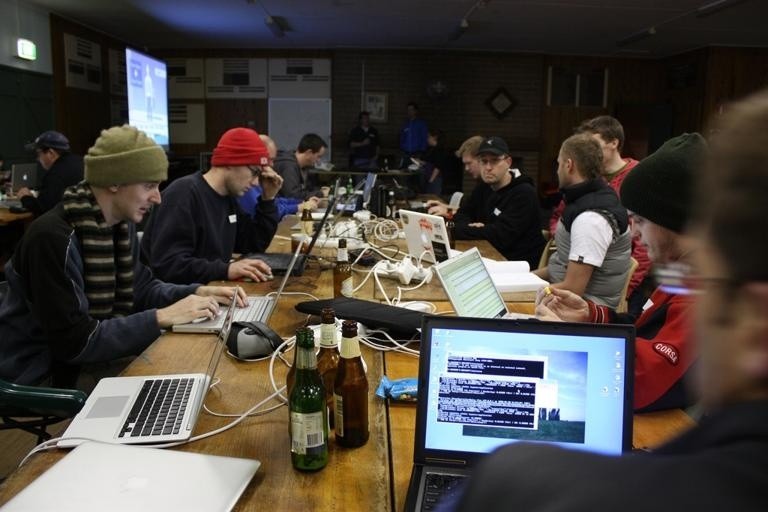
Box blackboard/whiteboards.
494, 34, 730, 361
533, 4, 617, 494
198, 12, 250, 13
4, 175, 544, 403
267, 98, 332, 163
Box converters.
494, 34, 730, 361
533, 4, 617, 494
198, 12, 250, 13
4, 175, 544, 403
395, 257, 416, 286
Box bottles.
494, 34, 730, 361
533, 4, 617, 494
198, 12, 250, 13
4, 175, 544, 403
333, 238, 353, 299
327, 172, 354, 205
445, 207, 456, 249
300, 205, 314, 237
286, 308, 370, 473
383, 157, 389, 173
385, 190, 397, 220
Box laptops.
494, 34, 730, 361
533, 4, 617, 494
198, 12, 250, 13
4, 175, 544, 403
336, 172, 377, 211
319, 177, 341, 201
432, 246, 537, 320
56, 286, 238, 449
339, 177, 366, 195
172, 234, 305, 335
1, 440, 261, 511
238, 197, 339, 276
391, 176, 439, 215
399, 209, 462, 264
402, 315, 635, 512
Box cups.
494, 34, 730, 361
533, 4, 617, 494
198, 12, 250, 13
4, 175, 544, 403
290, 233, 309, 256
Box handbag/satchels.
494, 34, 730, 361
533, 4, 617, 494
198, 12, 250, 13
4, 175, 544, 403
225, 320, 288, 356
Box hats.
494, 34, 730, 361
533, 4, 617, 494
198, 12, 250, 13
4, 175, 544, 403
83, 124, 170, 184
475, 136, 508, 156
620, 133, 714, 236
211, 127, 270, 166
26, 131, 70, 151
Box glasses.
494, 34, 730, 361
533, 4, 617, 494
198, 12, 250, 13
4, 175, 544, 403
248, 166, 261, 177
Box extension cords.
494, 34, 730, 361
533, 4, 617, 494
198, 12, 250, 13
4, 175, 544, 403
373, 261, 433, 284
307, 322, 365, 344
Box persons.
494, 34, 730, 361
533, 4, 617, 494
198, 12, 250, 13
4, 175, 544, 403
17, 130, 83, 219
235, 135, 321, 222
539, 132, 717, 415
273, 133, 331, 202
0, 125, 250, 417
139, 128, 285, 285
398, 101, 652, 314
350, 110, 382, 186
455, 93, 767, 512
143, 64, 153, 119
